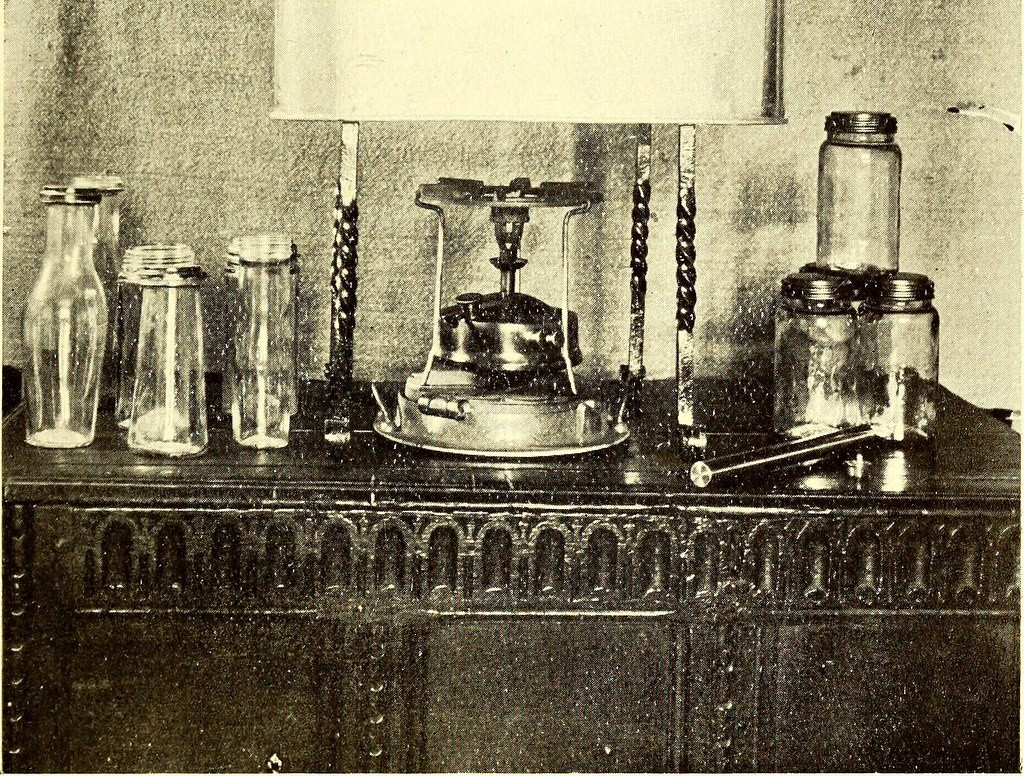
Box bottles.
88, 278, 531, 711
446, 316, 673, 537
128, 265, 209, 460
222, 238, 298, 418
20, 184, 108, 450
815, 111, 902, 278
772, 274, 858, 438
69, 174, 126, 410
231, 239, 296, 450
799, 262, 881, 329
114, 244, 196, 430
853, 271, 939, 441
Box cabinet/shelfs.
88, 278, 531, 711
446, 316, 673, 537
2, 384, 1021, 774
